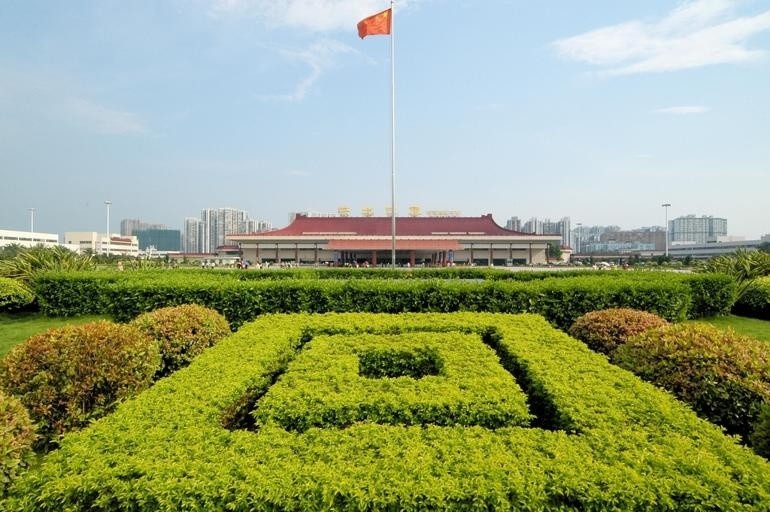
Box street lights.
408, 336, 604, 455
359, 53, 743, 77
104, 200, 113, 257
662, 203, 672, 257
27, 208, 37, 247
577, 222, 583, 254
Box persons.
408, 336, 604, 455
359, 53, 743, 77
236, 261, 290, 269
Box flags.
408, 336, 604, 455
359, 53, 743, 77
357, 8, 391, 40
